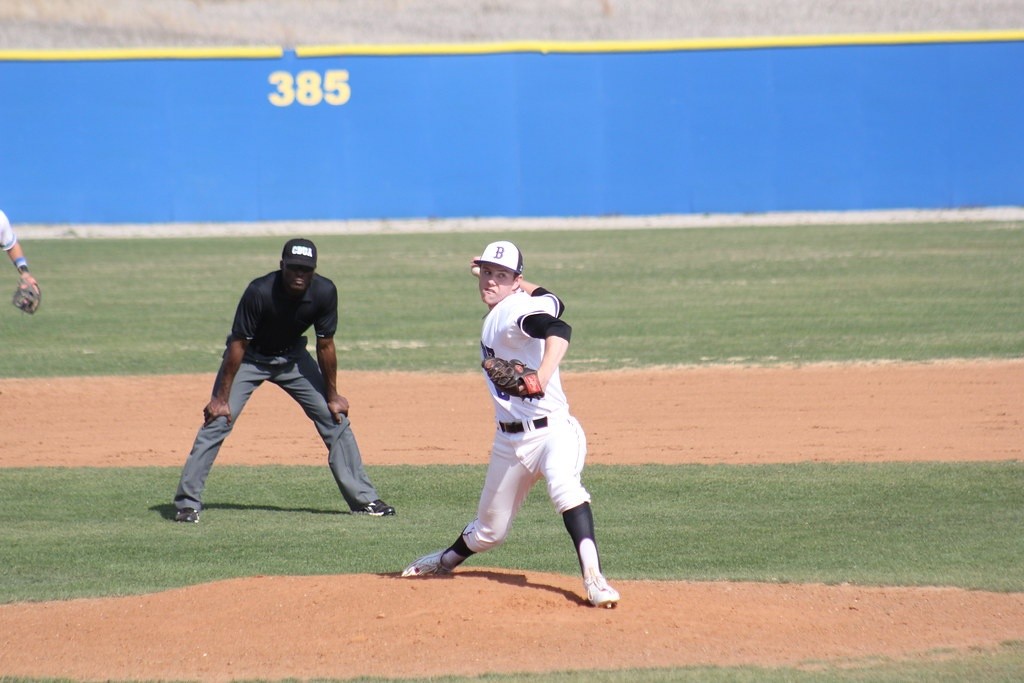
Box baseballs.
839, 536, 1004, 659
471, 264, 482, 278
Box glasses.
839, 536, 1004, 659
287, 265, 314, 272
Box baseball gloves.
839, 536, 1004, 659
13, 276, 41, 315
480, 354, 546, 401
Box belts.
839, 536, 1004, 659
499, 416, 548, 432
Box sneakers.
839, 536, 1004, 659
585, 574, 620, 608
351, 499, 395, 515
401, 549, 452, 576
175, 508, 200, 522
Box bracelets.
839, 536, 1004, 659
14, 257, 28, 269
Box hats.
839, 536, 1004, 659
474, 241, 523, 274
282, 238, 317, 268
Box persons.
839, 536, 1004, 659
400, 241, 620, 607
174, 239, 396, 523
0, 209, 40, 314
